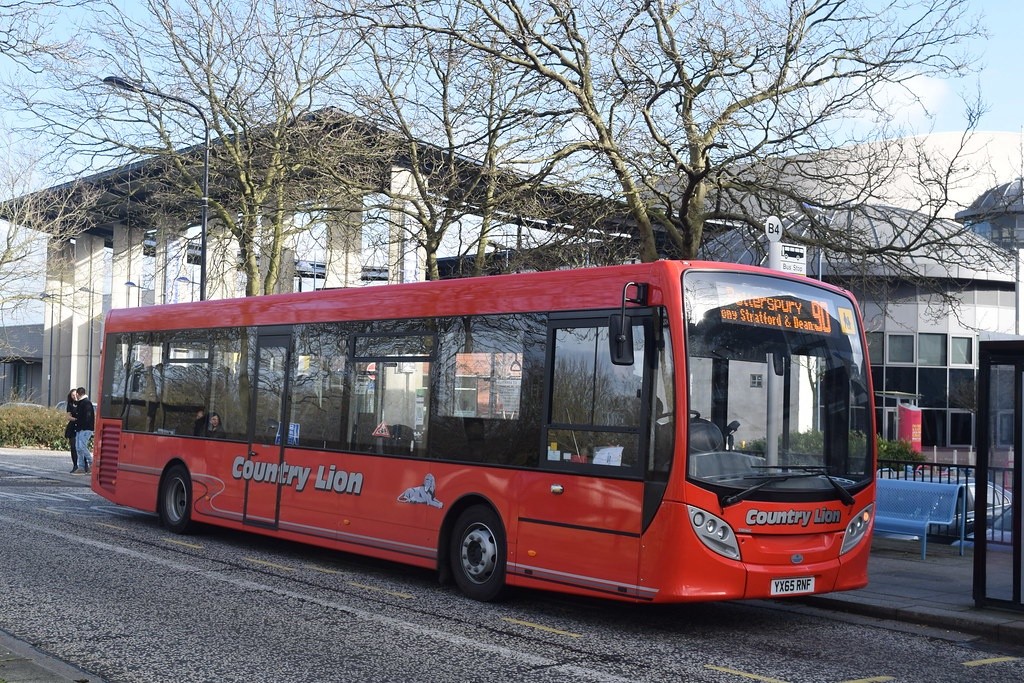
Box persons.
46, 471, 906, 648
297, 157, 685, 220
65, 388, 95, 474
192, 410, 227, 439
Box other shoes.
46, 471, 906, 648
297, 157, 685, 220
87, 466, 92, 474
70, 466, 78, 473
71, 468, 86, 475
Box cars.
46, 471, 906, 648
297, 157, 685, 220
952, 503, 1014, 553
866, 477, 1014, 546
55, 401, 96, 414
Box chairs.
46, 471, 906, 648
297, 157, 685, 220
257, 417, 280, 445
384, 423, 419, 457
636, 388, 664, 429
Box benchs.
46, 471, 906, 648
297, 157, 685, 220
871, 477, 967, 559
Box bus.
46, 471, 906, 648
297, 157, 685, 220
92, 259, 878, 605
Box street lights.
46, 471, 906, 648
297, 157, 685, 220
103, 77, 210, 300
41, 293, 54, 408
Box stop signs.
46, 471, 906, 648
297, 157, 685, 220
367, 363, 376, 380
